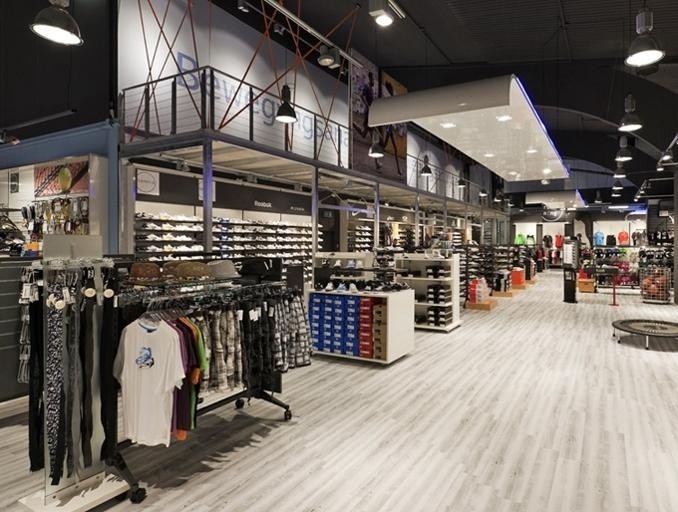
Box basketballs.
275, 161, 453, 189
639, 268, 670, 300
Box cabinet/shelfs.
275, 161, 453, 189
135, 200, 516, 364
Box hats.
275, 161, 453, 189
237, 260, 273, 275
124, 263, 167, 286
206, 260, 242, 280
160, 262, 215, 285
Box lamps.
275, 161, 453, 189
613, 1, 666, 191
30, 1, 85, 48
274, 0, 342, 123
368, 22, 468, 187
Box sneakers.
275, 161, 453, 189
354, 225, 371, 252
281, 265, 313, 279
134, 212, 323, 264
316, 259, 409, 293
